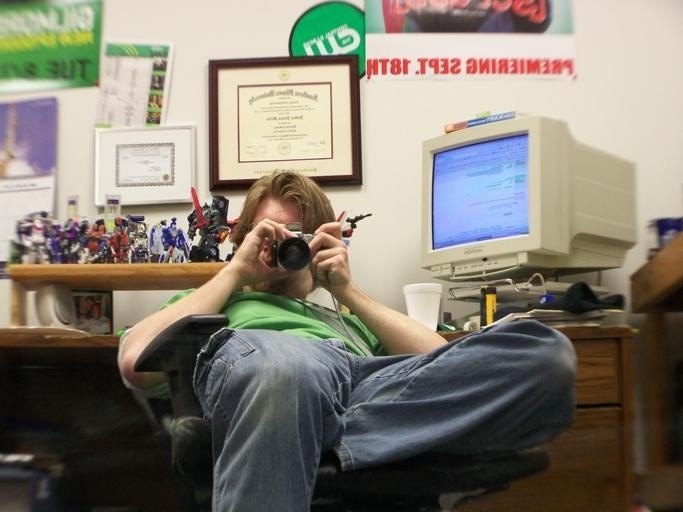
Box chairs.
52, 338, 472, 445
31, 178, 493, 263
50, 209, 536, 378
132, 388, 432, 511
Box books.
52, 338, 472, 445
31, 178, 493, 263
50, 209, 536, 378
444, 108, 527, 134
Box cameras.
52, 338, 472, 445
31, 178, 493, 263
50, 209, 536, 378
272, 223, 316, 272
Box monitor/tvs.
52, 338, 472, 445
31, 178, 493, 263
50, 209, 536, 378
419, 114, 639, 302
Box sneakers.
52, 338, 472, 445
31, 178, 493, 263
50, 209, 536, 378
169, 414, 214, 491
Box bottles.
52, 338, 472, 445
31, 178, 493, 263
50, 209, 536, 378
480, 286, 496, 328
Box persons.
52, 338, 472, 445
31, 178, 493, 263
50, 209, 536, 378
15, 187, 240, 265
116, 167, 578, 512
77, 296, 111, 334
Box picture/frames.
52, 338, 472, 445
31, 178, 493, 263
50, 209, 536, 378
70, 289, 113, 334
209, 54, 362, 191
95, 125, 197, 206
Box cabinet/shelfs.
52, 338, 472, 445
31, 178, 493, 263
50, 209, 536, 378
434, 325, 641, 512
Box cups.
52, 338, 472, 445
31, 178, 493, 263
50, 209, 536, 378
403, 283, 441, 332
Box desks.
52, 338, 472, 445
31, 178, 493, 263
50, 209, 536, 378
0, 325, 120, 350
629, 231, 683, 512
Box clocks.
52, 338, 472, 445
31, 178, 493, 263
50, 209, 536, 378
35, 282, 71, 329
